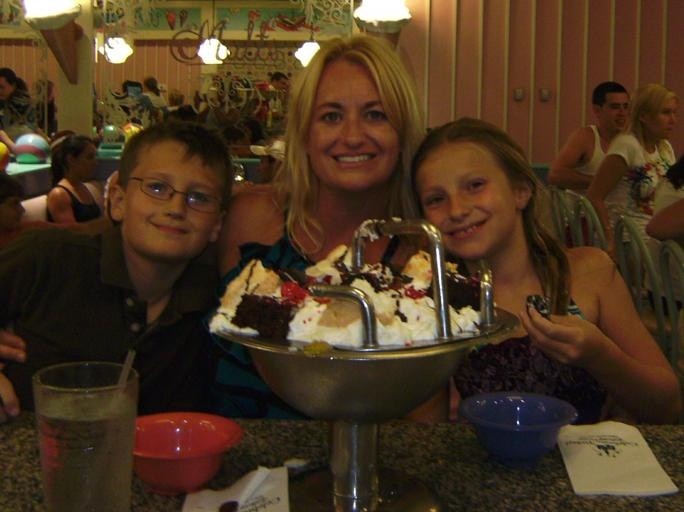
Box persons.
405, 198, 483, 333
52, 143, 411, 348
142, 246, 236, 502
0, 170, 107, 247
582, 82, 681, 266
411, 118, 684, 426
0, 67, 289, 157
47, 134, 106, 225
642, 155, 683, 318
0, 119, 307, 418
0, 27, 450, 424
547, 82, 630, 248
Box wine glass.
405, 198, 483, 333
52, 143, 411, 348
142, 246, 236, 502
204, 306, 521, 512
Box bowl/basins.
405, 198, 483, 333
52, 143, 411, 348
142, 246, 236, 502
457, 390, 580, 464
129, 410, 245, 496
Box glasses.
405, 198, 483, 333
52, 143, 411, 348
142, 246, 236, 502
128, 176, 225, 216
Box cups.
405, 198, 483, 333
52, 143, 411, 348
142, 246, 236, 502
30, 360, 140, 511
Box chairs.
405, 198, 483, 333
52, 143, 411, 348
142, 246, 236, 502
548, 187, 684, 384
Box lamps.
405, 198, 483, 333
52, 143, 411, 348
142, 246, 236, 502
295, 22, 321, 67
197, 0, 231, 65
352, 0, 412, 25
